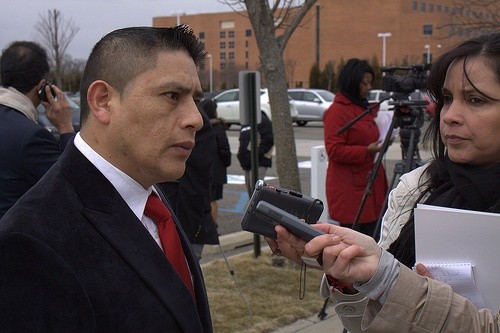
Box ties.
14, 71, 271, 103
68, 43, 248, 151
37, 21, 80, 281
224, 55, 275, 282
143, 189, 194, 301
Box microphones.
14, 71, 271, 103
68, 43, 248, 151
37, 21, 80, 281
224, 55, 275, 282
412, 63, 433, 73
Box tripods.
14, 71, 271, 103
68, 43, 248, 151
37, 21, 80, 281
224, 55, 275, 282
317, 93, 429, 333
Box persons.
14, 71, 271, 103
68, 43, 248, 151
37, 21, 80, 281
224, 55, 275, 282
263, 30, 499, 333
2, 25, 214, 333
0, 41, 79, 219
157, 97, 274, 263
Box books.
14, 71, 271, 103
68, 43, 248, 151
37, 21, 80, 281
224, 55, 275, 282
411, 203, 500, 315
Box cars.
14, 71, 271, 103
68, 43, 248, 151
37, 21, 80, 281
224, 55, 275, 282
35, 94, 80, 133
287, 89, 338, 125
366, 90, 396, 112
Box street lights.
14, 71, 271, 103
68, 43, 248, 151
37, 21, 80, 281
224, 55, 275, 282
173, 12, 186, 25
424, 45, 431, 78
378, 32, 392, 77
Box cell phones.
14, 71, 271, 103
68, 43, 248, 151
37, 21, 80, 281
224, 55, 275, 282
37, 83, 56, 103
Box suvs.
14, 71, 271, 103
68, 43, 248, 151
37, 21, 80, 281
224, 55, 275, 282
211, 88, 299, 129
